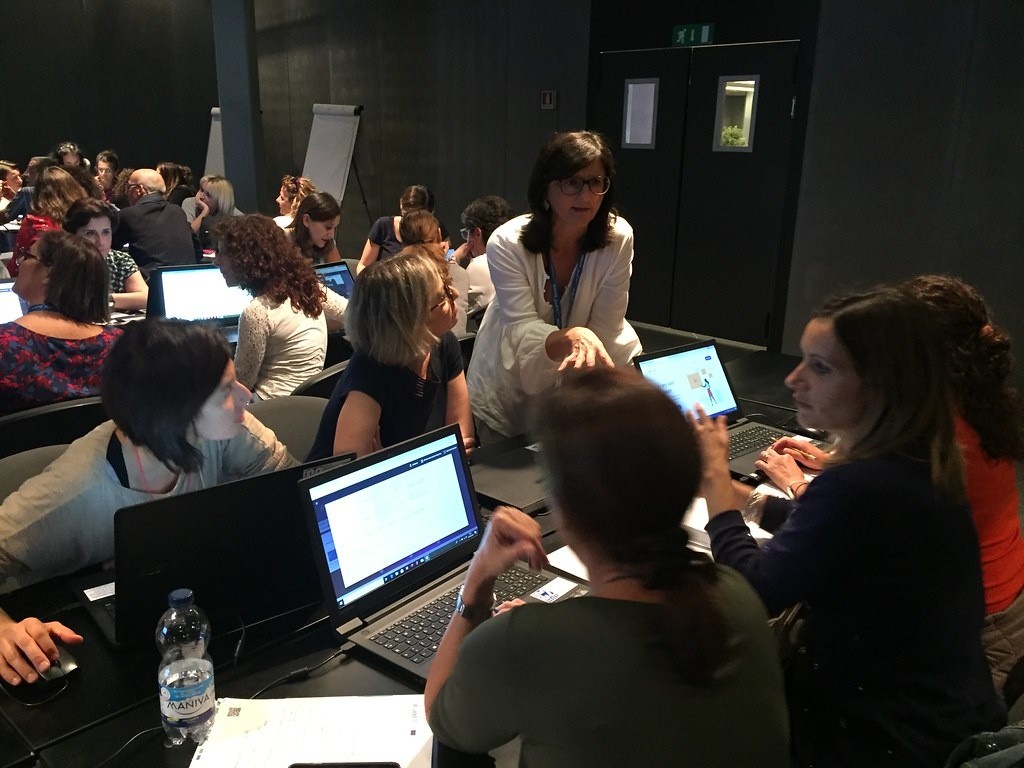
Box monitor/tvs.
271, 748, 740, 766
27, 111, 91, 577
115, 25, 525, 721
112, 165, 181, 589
0, 278, 30, 323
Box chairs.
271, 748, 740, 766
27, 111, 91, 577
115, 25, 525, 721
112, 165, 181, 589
1, 303, 489, 505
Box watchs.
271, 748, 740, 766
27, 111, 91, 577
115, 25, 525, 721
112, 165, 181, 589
107, 294, 115, 308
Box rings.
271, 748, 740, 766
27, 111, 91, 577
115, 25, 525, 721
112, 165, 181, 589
763, 453, 770, 461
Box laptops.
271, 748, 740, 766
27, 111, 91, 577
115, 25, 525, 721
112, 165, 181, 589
310, 260, 355, 299
445, 246, 456, 260
78, 422, 592, 681
632, 339, 797, 487
114, 262, 253, 345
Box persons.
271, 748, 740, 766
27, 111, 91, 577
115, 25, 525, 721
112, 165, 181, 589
0, 317, 303, 684
302, 246, 474, 461
0, 142, 197, 225
111, 168, 200, 286
355, 186, 433, 273
422, 370, 796, 768
396, 196, 512, 341
281, 191, 341, 265
273, 175, 316, 235
685, 285, 1003, 768
7, 167, 90, 278
752, 275, 1023, 695
62, 199, 148, 311
210, 211, 348, 406
181, 175, 247, 246
464, 130, 642, 446
0, 231, 121, 416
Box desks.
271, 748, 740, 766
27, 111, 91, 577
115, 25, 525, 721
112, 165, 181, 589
1, 351, 804, 768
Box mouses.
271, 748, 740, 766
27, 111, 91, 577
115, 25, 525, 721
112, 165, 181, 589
27, 644, 80, 686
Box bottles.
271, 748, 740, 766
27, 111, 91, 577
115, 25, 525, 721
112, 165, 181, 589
155, 588, 217, 745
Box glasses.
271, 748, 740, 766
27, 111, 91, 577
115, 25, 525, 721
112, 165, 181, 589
460, 224, 471, 240
124, 181, 142, 191
555, 176, 611, 195
15, 246, 52, 267
432, 282, 453, 310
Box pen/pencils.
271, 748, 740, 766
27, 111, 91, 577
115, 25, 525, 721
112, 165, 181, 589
771, 436, 816, 461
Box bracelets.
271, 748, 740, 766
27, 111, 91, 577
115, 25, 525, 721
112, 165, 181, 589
786, 480, 809, 499
456, 585, 496, 621
